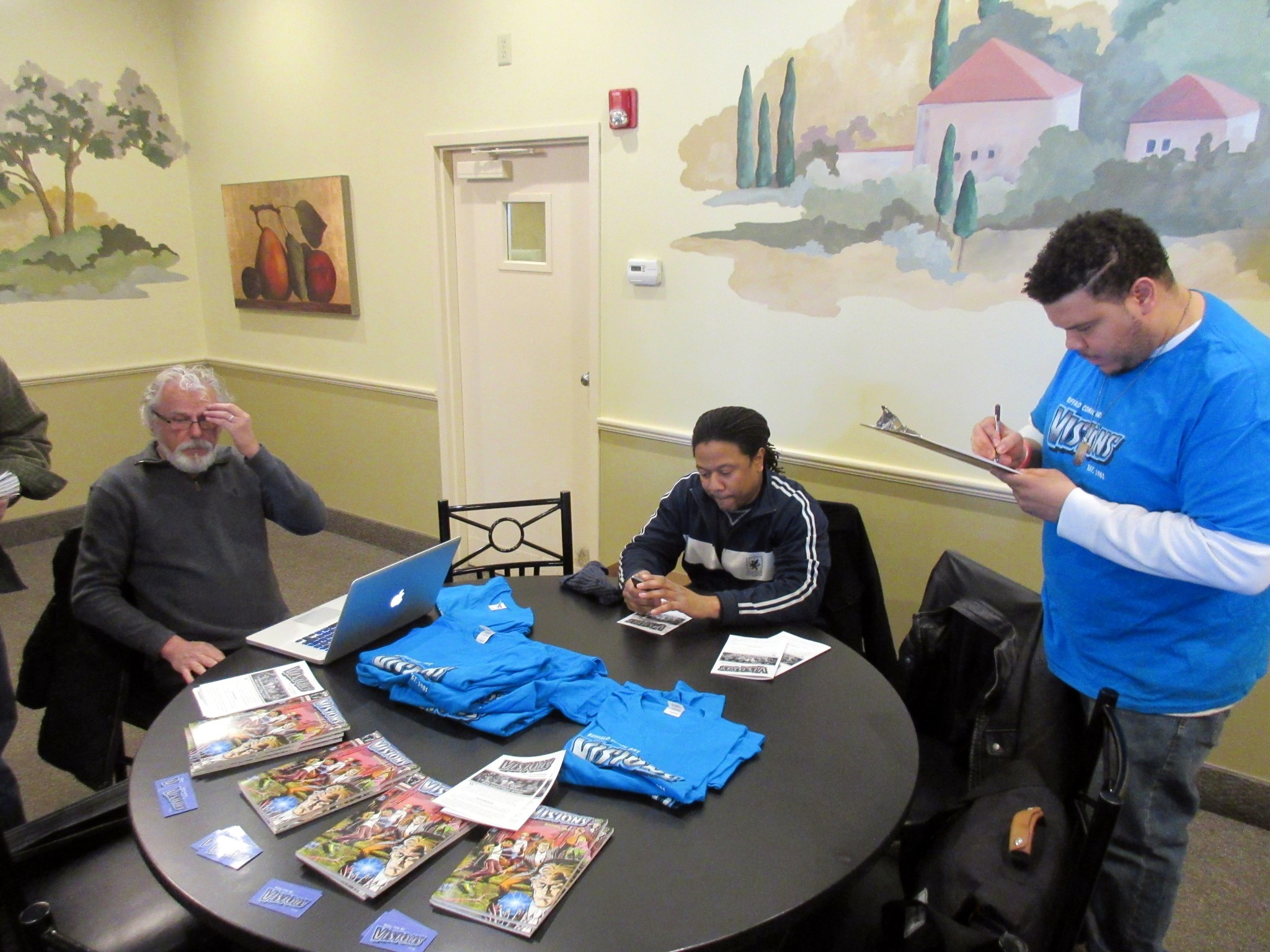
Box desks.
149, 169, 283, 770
122, 571, 926, 952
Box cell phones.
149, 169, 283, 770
631, 576, 653, 591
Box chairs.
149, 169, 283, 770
0, 488, 1137, 952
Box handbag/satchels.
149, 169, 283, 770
905, 778, 1068, 951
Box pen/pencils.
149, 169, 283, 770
994, 404, 1000, 462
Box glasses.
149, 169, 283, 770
151, 410, 219, 430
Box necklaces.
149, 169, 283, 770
1074, 288, 1192, 466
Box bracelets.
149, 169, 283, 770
1015, 439, 1032, 469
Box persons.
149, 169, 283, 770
459, 826, 589, 925
613, 409, 830, 632
969, 209, 1270, 952
202, 710, 323, 767
67, 365, 326, 685
322, 795, 456, 893
260, 756, 392, 833
0, 357, 67, 830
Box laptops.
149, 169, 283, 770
246, 536, 461, 665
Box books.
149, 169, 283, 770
184, 690, 615, 938
